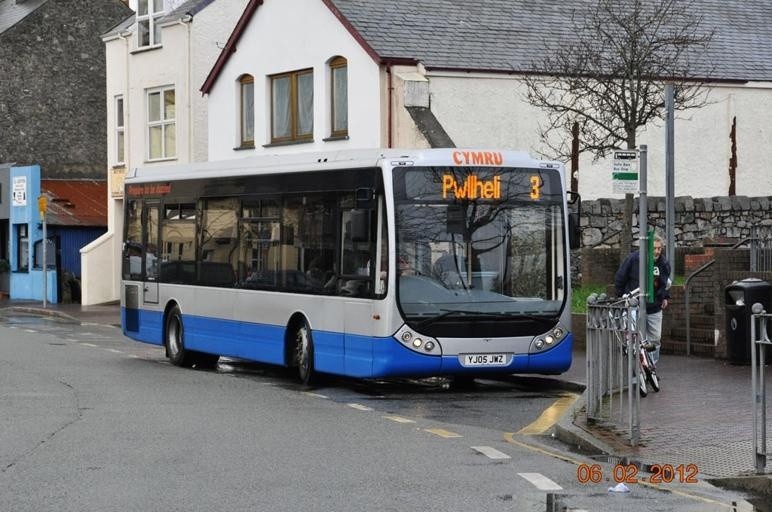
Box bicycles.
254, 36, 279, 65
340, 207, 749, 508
597, 287, 660, 397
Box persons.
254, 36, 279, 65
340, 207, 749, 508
616, 234, 671, 367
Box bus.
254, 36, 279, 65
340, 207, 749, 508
120, 149, 573, 385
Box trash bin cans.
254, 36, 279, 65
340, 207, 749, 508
724, 277, 772, 366
